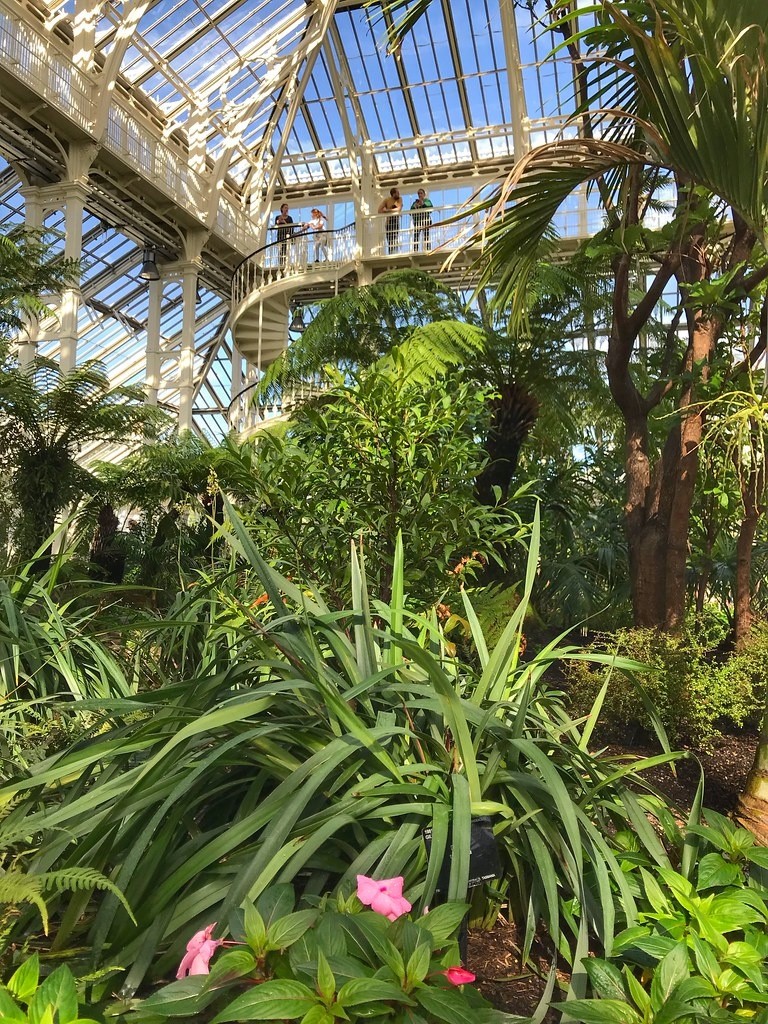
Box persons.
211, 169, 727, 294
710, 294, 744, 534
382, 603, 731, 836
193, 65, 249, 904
275, 204, 293, 267
378, 187, 403, 255
408, 188, 433, 251
302, 208, 329, 263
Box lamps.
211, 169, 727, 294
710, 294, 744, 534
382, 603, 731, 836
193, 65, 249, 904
137, 245, 160, 280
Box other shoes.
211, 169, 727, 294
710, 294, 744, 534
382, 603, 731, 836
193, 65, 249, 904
314, 260, 320, 263
323, 259, 331, 262
